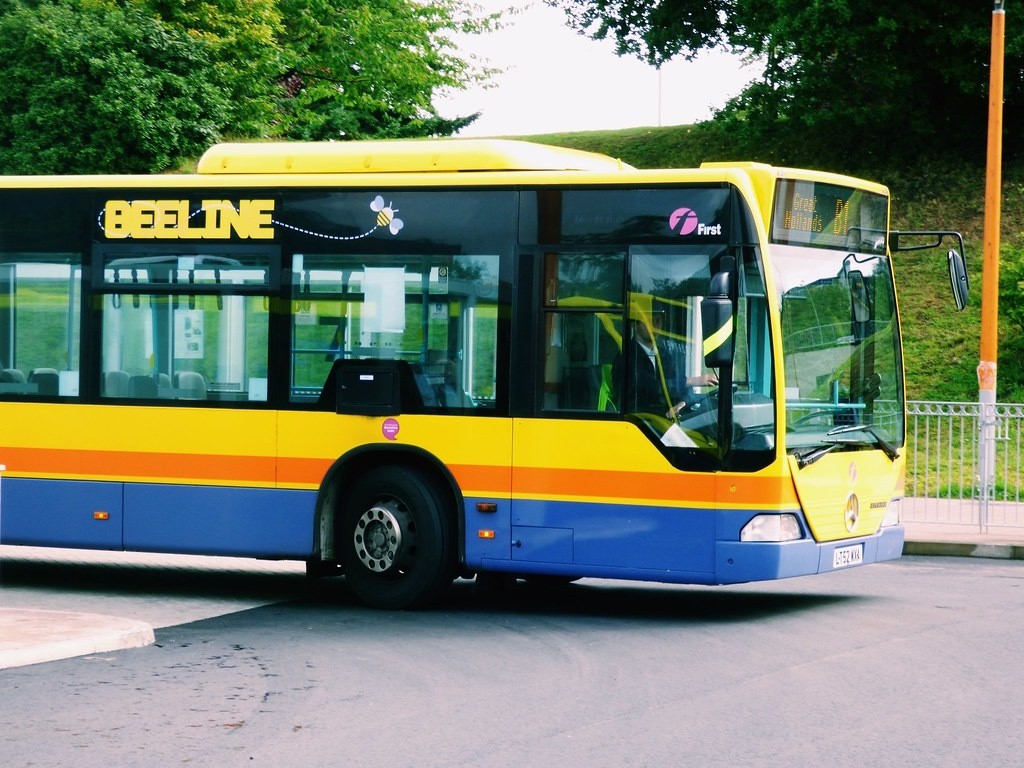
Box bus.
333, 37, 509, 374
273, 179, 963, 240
0, 139, 971, 610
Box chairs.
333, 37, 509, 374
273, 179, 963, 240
590, 365, 618, 412
0, 368, 207, 402
314, 358, 461, 410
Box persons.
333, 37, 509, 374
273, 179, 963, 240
612, 311, 719, 419
